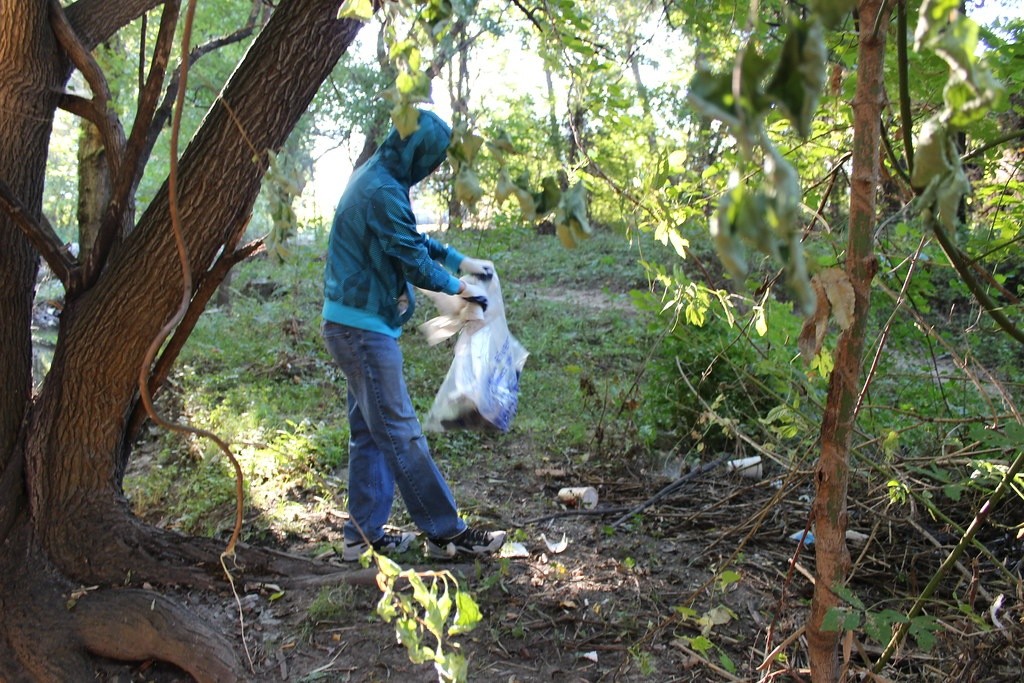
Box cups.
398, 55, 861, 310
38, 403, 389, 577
727, 456, 763, 480
559, 487, 598, 511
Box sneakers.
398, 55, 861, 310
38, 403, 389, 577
343, 526, 417, 563
424, 526, 508, 561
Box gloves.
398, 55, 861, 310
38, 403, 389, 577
461, 256, 494, 280
458, 279, 488, 314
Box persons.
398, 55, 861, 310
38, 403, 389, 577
322, 109, 506, 561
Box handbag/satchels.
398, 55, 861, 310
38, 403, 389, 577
424, 274, 531, 435
